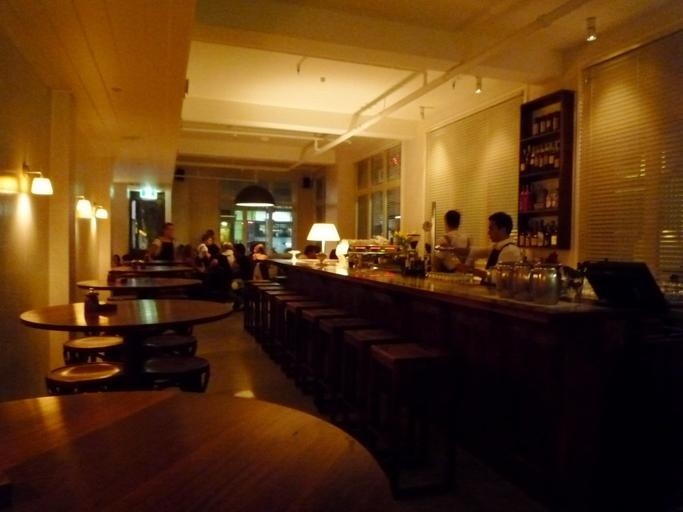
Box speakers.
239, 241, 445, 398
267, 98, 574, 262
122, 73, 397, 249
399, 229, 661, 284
303, 177, 310, 187
175, 168, 184, 181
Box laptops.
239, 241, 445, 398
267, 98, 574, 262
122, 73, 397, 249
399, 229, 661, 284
581, 261, 683, 309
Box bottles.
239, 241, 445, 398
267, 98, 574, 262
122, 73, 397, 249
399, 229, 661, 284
518, 108, 561, 250
85, 286, 100, 314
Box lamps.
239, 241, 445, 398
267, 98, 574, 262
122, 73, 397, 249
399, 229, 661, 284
70, 192, 94, 220
583, 16, 600, 43
91, 199, 109, 220
231, 162, 276, 209
16, 159, 53, 199
303, 222, 340, 255
472, 74, 483, 96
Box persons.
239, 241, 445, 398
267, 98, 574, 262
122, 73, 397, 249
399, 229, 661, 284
430, 209, 471, 275
452, 212, 521, 288
146, 222, 177, 262
196, 228, 269, 312
298, 245, 316, 260
314, 246, 322, 258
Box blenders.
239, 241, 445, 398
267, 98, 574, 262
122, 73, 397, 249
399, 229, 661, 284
404, 232, 432, 276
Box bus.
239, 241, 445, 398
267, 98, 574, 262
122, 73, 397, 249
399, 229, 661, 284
244, 220, 291, 254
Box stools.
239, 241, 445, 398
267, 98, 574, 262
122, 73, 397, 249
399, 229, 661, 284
244, 279, 386, 403
64, 336, 124, 364
369, 342, 445, 442
45, 362, 123, 394
143, 331, 198, 355
343, 329, 401, 424
140, 355, 210, 394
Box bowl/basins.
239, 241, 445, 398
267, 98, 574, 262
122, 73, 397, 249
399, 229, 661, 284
355, 245, 396, 252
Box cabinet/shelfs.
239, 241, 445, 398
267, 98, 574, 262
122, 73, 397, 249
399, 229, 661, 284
515, 87, 573, 250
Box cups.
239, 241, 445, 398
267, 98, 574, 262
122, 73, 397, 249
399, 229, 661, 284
489, 260, 568, 308
